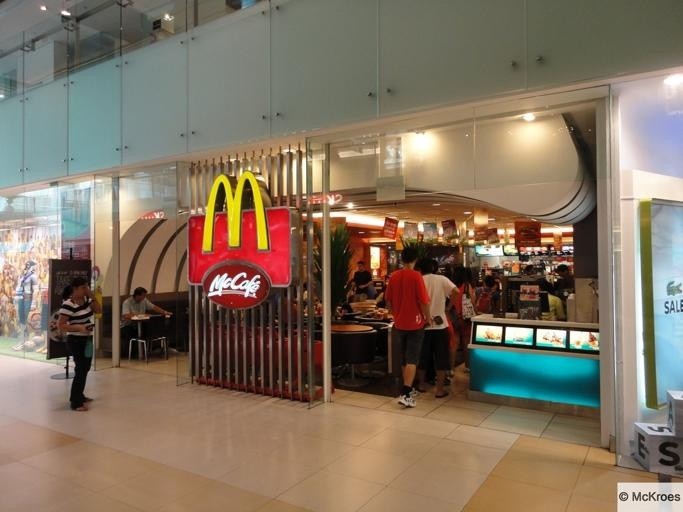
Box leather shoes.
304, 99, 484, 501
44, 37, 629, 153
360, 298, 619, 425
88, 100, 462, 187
69, 396, 93, 411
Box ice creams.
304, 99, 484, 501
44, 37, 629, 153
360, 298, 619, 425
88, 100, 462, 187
588, 332, 599, 348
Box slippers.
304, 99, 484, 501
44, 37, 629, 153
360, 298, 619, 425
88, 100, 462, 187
435, 391, 449, 398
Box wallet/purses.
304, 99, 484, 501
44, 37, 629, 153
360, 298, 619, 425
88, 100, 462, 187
85, 324, 96, 331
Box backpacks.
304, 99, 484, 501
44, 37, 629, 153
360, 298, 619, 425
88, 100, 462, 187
475, 287, 496, 315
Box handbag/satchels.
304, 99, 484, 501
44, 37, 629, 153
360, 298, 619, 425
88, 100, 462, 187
461, 282, 477, 320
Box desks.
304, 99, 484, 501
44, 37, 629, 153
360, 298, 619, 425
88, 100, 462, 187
131, 314, 153, 362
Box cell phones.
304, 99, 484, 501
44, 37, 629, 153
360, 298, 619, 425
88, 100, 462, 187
86, 324, 95, 330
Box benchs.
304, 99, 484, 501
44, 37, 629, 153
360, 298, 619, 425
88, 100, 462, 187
104, 293, 187, 356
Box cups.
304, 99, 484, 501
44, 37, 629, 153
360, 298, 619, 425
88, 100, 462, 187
374, 308, 388, 321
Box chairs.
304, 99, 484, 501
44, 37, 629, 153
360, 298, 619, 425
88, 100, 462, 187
126, 313, 171, 363
289, 291, 471, 395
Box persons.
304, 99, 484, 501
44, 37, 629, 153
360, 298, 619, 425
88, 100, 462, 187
14, 260, 38, 351
120, 287, 173, 360
57, 277, 102, 411
40, 284, 48, 354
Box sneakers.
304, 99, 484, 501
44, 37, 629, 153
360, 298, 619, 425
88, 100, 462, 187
397, 394, 416, 408
412, 369, 454, 393
399, 387, 418, 398
